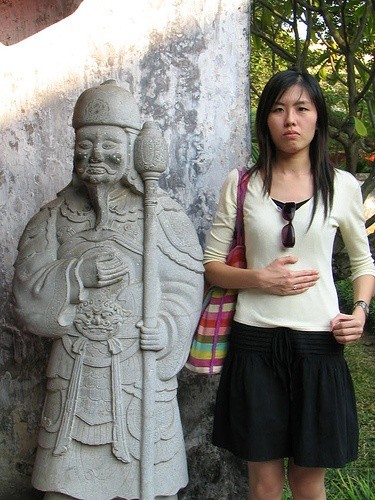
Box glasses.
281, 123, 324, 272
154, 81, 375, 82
281, 201, 296, 248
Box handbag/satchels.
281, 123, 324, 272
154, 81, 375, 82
183, 166, 252, 375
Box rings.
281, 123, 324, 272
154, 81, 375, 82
293, 285, 297, 289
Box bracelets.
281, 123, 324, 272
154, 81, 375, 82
353, 300, 369, 318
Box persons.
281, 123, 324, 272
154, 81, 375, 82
13, 79, 206, 500
205, 70, 375, 500
